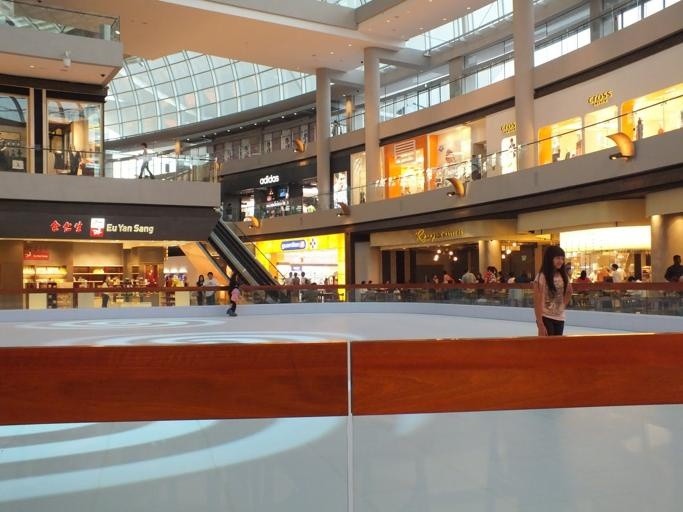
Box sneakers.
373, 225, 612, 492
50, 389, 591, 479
227, 308, 237, 316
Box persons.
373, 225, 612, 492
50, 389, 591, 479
636, 118, 643, 141
137, 143, 154, 180
284, 138, 290, 150
285, 262, 649, 315
551, 135, 560, 163
0, 133, 7, 170
664, 255, 682, 309
303, 134, 308, 144
243, 145, 249, 158
202, 272, 219, 305
267, 142, 270, 153
533, 246, 572, 337
226, 273, 238, 315
165, 275, 172, 288
183, 276, 188, 287
332, 120, 338, 137
196, 275, 204, 305
100, 275, 110, 308
337, 122, 342, 136
470, 154, 481, 180
228, 281, 239, 317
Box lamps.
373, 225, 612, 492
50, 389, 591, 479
432, 246, 459, 264
445, 177, 466, 199
605, 132, 635, 161
292, 138, 305, 153
336, 202, 351, 217
501, 242, 523, 260
247, 216, 260, 229
